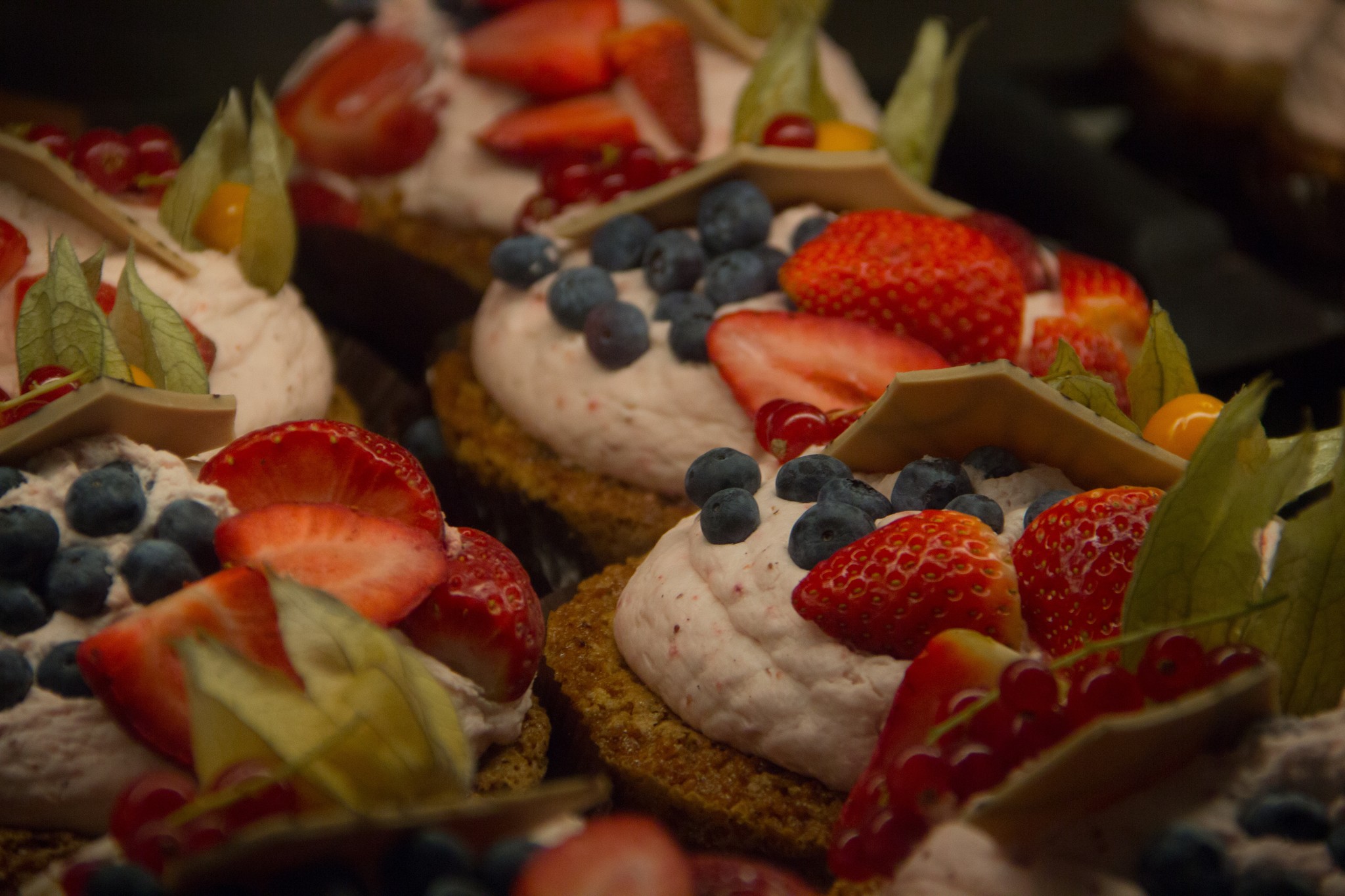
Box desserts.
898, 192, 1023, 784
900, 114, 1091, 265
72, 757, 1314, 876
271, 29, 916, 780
0, 0, 1345, 896
